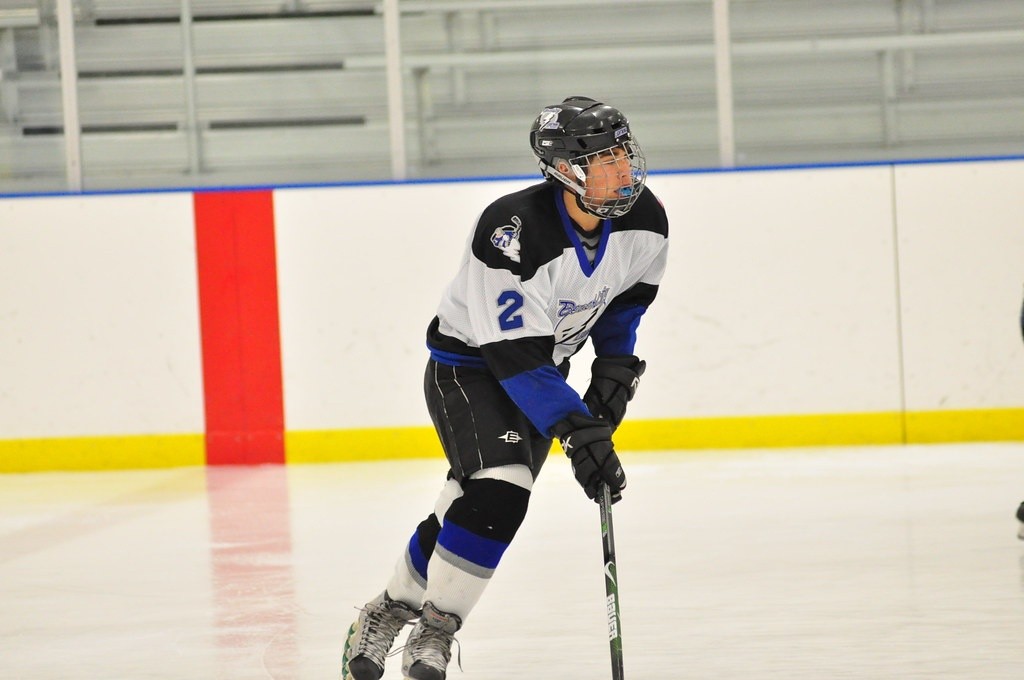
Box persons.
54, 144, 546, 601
341, 93, 673, 680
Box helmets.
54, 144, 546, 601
529, 96, 647, 219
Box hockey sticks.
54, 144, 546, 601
591, 473, 634, 680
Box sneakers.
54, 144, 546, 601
386, 601, 463, 680
342, 588, 423, 680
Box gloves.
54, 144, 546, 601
583, 355, 646, 435
553, 412, 627, 505
